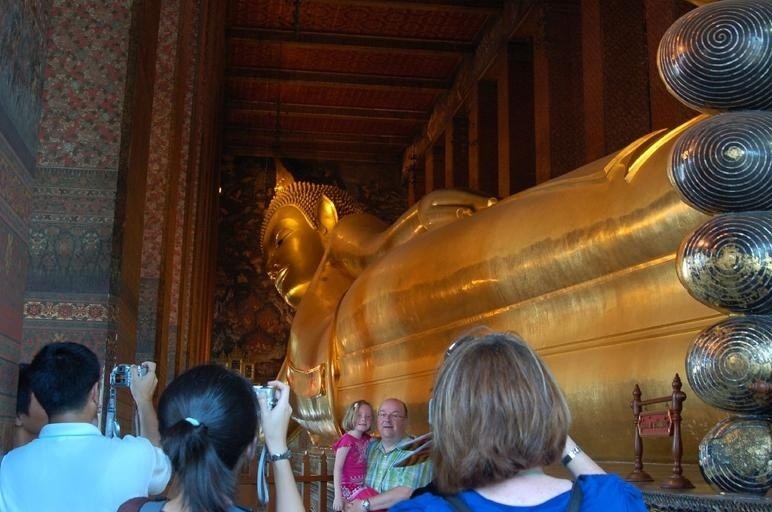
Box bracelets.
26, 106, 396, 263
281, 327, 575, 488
560, 446, 582, 467
269, 449, 293, 463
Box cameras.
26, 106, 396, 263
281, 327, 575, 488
252, 384, 276, 411
109, 363, 149, 388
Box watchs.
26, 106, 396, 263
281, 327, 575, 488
362, 497, 371, 512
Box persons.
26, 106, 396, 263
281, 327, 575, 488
116, 363, 307, 512
257, 177, 495, 435
386, 329, 648, 512
332, 400, 386, 511
0, 341, 172, 512
13, 362, 50, 450
341, 398, 434, 512
